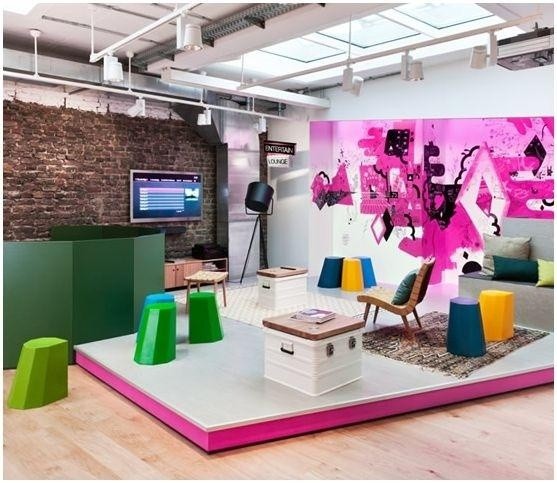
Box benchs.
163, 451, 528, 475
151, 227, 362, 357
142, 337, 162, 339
458, 215, 554, 336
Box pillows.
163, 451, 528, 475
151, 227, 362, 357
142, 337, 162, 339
533, 260, 554, 287
474, 229, 531, 275
489, 251, 539, 284
389, 267, 418, 306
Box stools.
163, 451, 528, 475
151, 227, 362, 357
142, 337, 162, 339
182, 268, 230, 313
7, 336, 71, 411
444, 295, 486, 357
135, 291, 178, 344
475, 288, 515, 342
186, 290, 223, 343
131, 302, 180, 366
318, 252, 377, 292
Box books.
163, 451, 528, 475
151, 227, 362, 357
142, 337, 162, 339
288, 307, 336, 323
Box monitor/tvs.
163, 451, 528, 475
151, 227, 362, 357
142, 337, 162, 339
129, 169, 203, 223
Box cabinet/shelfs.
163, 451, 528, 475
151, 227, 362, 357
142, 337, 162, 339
255, 264, 308, 311
165, 255, 229, 289
260, 307, 366, 397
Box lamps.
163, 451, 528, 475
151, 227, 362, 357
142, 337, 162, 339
400, 55, 424, 81
342, 65, 364, 97
238, 181, 277, 284
176, 15, 203, 53
470, 31, 499, 70
197, 110, 212, 125
104, 51, 123, 82
254, 118, 266, 132
127, 97, 145, 118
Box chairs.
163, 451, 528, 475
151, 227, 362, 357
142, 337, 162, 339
356, 255, 440, 338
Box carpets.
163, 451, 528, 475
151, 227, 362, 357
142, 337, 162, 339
358, 310, 554, 378
175, 282, 381, 330
